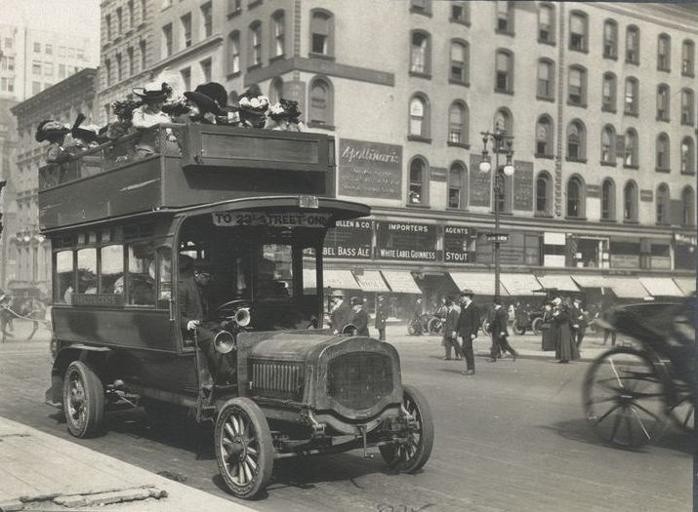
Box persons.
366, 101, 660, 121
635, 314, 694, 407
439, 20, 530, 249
415, 294, 622, 365
62, 248, 171, 313
451, 288, 479, 376
374, 294, 389, 341
347, 291, 372, 337
245, 257, 292, 303
327, 288, 353, 335
33, 79, 301, 166
173, 255, 236, 386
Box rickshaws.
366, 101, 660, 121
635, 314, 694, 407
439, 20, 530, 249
580, 301, 697, 450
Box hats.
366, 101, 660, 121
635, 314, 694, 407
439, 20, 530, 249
331, 290, 342, 297
350, 297, 361, 305
192, 259, 213, 271
460, 290, 474, 295
111, 81, 301, 120
444, 296, 455, 305
35, 119, 70, 141
71, 124, 100, 141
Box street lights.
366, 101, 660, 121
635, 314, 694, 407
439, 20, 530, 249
478, 121, 516, 298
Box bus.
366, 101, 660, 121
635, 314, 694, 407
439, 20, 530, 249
37, 121, 436, 501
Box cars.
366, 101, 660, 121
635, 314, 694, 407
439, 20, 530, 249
511, 287, 587, 337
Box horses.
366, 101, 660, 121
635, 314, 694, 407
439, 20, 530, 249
0, 287, 51, 344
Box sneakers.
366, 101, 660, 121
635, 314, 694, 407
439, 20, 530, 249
443, 352, 463, 360
489, 351, 517, 362
462, 369, 475, 374
